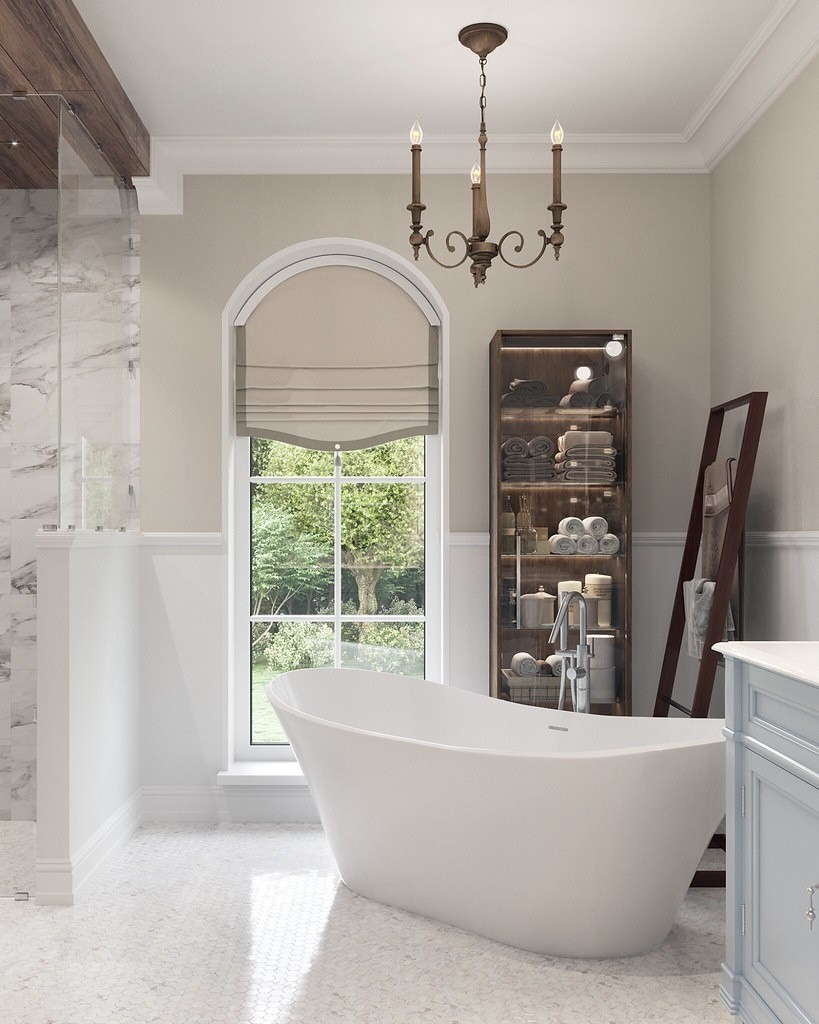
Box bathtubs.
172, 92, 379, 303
266, 668, 726, 959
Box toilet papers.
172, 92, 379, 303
587, 635, 614, 668
589, 668, 616, 703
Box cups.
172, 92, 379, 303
572, 587, 601, 629
533, 585, 557, 629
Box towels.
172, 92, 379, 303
501, 378, 557, 407
701, 456, 737, 582
536, 659, 552, 674
501, 436, 558, 482
555, 431, 617, 482
559, 379, 618, 408
545, 655, 570, 676
510, 652, 540, 676
548, 517, 620, 555
683, 578, 736, 667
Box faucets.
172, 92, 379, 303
548, 591, 596, 713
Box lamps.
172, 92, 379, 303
407, 22, 569, 289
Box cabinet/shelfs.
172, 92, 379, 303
712, 640, 819, 1024
488, 329, 633, 724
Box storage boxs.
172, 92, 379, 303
500, 668, 578, 703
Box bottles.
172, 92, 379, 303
501, 493, 515, 555
516, 496, 531, 555
